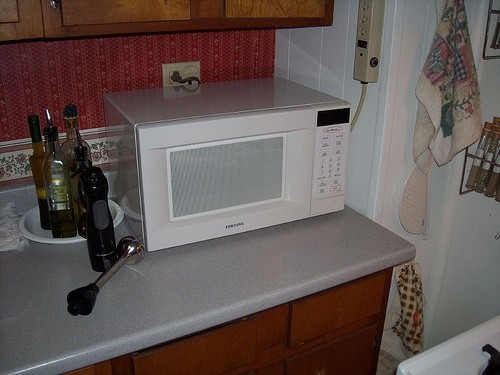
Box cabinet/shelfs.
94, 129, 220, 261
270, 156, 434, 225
15, 339, 121, 375
121, 243, 415, 375
41, 0, 333, 40
0, 0, 43, 44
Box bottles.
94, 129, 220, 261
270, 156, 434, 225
84, 165, 118, 272
59, 105, 92, 240
39, 126, 78, 238
28, 116, 53, 230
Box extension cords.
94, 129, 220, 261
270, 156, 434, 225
352, 1, 387, 84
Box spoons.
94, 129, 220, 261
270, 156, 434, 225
66, 236, 144, 316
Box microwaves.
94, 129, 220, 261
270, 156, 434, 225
101, 74, 354, 255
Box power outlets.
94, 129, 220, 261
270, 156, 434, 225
161, 61, 200, 88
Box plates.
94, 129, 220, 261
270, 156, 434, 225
17, 199, 127, 246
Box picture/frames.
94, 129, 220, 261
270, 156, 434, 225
484, 1, 500, 60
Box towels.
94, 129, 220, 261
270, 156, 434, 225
414, 1, 482, 175
395, 262, 423, 357
